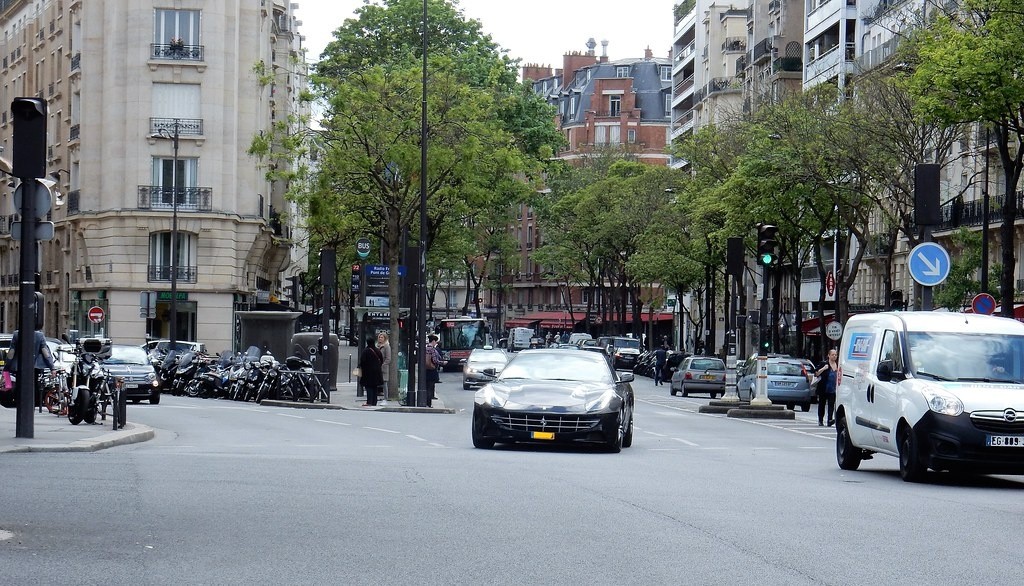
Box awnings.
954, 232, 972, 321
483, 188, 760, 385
506, 319, 537, 327
540, 320, 580, 328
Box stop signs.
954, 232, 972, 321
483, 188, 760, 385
88, 306, 105, 323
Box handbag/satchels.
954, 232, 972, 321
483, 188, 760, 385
425, 348, 435, 371
2, 370, 12, 389
353, 366, 362, 378
811, 375, 822, 387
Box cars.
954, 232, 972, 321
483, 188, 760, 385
499, 337, 508, 350
550, 331, 642, 370
669, 356, 728, 397
97, 345, 162, 405
734, 352, 821, 414
471, 347, 635, 454
649, 350, 694, 380
138, 338, 207, 355
0, 332, 80, 409
459, 346, 510, 389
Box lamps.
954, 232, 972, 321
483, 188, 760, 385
48, 169, 69, 181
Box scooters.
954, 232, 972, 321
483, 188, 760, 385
57, 333, 114, 426
147, 345, 321, 405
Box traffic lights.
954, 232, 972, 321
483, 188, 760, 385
756, 223, 780, 265
283, 276, 300, 302
762, 327, 771, 351
890, 288, 904, 313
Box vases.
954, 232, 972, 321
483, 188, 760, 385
169, 46, 184, 51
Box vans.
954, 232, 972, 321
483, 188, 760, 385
831, 309, 1024, 486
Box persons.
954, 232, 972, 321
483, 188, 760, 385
360, 337, 384, 406
426, 335, 448, 407
815, 349, 838, 426
655, 345, 665, 386
377, 334, 391, 400
3, 330, 56, 387
662, 335, 669, 349
546, 332, 572, 348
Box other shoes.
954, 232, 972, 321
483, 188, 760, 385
660, 382, 663, 385
656, 382, 658, 386
362, 404, 372, 406
828, 418, 835, 426
432, 396, 438, 400
819, 421, 823, 426
434, 381, 442, 383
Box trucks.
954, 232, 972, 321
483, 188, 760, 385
505, 327, 534, 353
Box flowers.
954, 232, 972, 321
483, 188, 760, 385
170, 37, 183, 46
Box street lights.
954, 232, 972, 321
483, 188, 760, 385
153, 116, 181, 350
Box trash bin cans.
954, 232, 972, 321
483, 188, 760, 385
399, 369, 408, 398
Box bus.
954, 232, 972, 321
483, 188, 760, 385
434, 316, 492, 373
